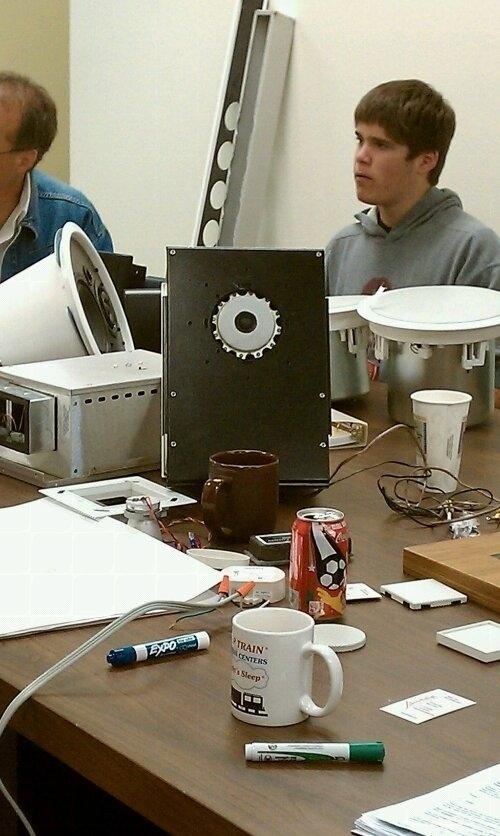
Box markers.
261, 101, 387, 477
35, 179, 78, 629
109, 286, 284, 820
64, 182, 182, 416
105, 632, 209, 670
242, 739, 385, 766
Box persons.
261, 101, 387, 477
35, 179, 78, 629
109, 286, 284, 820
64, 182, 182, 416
323, 79, 500, 427
0, 69, 115, 287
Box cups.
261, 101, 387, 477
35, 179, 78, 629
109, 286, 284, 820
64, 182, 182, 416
409, 389, 473, 493
229, 606, 345, 727
201, 450, 280, 541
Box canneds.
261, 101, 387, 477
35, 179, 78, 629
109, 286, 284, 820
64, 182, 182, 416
287, 508, 348, 622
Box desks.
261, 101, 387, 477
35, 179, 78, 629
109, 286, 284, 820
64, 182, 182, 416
1, 379, 500, 836
